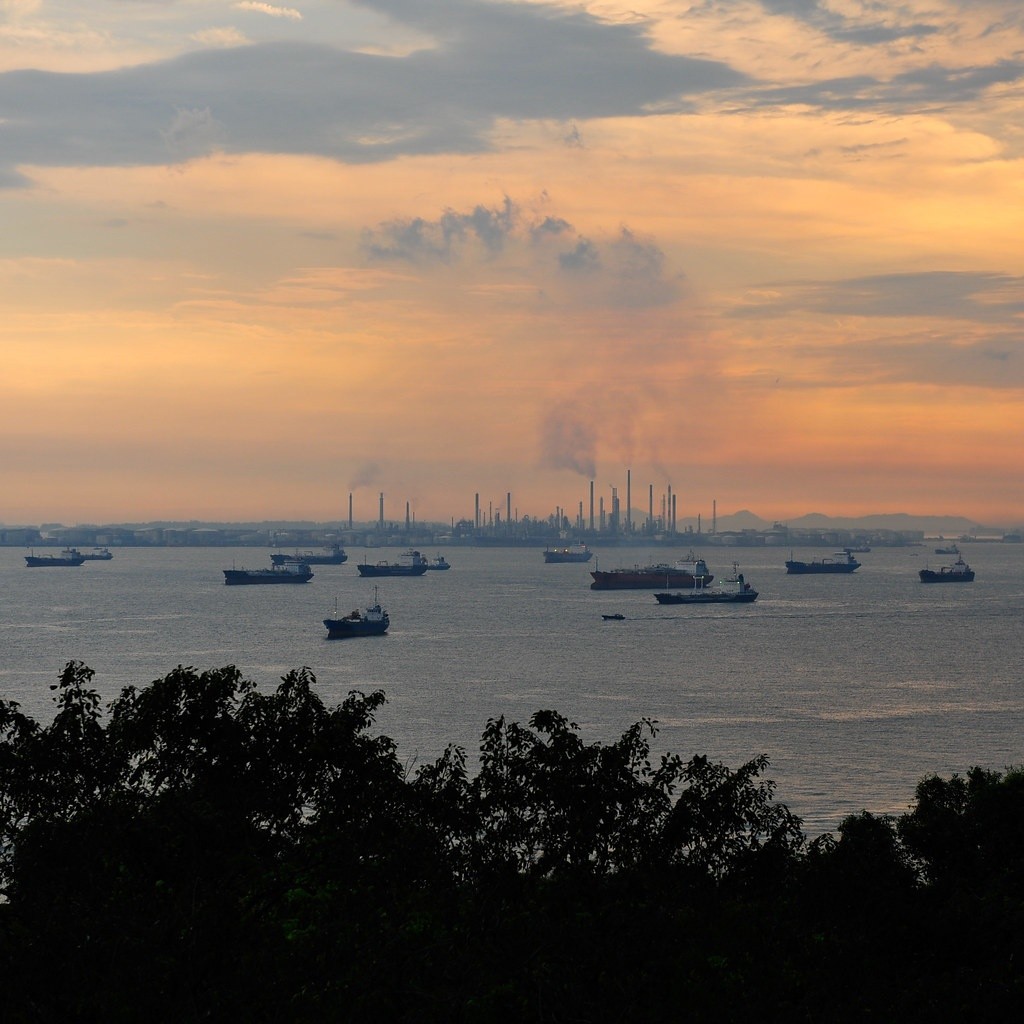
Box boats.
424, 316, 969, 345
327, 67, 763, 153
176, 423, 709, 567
590, 559, 713, 588
935, 545, 959, 554
270, 547, 348, 565
835, 552, 848, 555
23, 545, 86, 567
676, 553, 702, 565
602, 613, 625, 620
357, 551, 429, 577
843, 544, 871, 552
918, 564, 974, 582
223, 558, 315, 585
427, 554, 451, 570
323, 581, 390, 639
653, 573, 758, 604
785, 549, 861, 573
81, 549, 112, 560
542, 545, 593, 562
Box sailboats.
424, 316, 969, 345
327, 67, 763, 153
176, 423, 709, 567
955, 554, 965, 565
